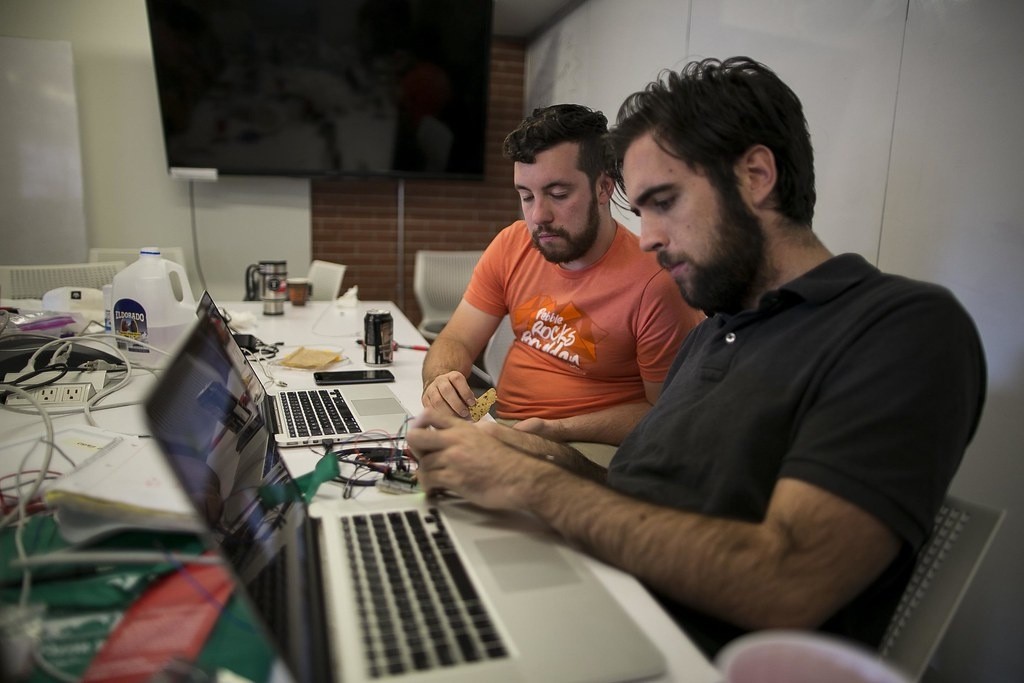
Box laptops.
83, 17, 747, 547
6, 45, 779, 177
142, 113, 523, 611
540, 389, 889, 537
141, 313, 668, 682
195, 290, 416, 448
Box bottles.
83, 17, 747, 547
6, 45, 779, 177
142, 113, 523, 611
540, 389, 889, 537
246, 260, 289, 316
102, 284, 112, 334
110, 246, 197, 369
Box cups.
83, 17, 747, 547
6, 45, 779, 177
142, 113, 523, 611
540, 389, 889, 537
723, 637, 902, 682
288, 281, 309, 306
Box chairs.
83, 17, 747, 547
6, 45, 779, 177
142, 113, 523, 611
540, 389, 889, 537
878, 498, 1004, 683
0, 247, 186, 301
483, 313, 516, 388
413, 251, 490, 384
307, 260, 347, 301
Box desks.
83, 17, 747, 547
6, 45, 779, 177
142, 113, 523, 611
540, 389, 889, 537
0, 301, 722, 683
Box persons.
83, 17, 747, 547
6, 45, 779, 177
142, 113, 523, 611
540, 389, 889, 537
406, 54, 987, 660
421, 104, 706, 443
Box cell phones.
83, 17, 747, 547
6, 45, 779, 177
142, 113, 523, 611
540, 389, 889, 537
313, 369, 395, 386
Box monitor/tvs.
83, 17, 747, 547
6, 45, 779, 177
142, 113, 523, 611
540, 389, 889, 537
145, 0, 494, 179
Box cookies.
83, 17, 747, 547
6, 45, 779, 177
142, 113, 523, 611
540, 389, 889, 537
468, 388, 497, 422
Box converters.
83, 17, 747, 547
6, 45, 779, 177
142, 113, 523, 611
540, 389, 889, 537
231, 333, 269, 356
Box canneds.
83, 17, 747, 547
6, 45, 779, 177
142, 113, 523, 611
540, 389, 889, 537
362, 308, 393, 366
